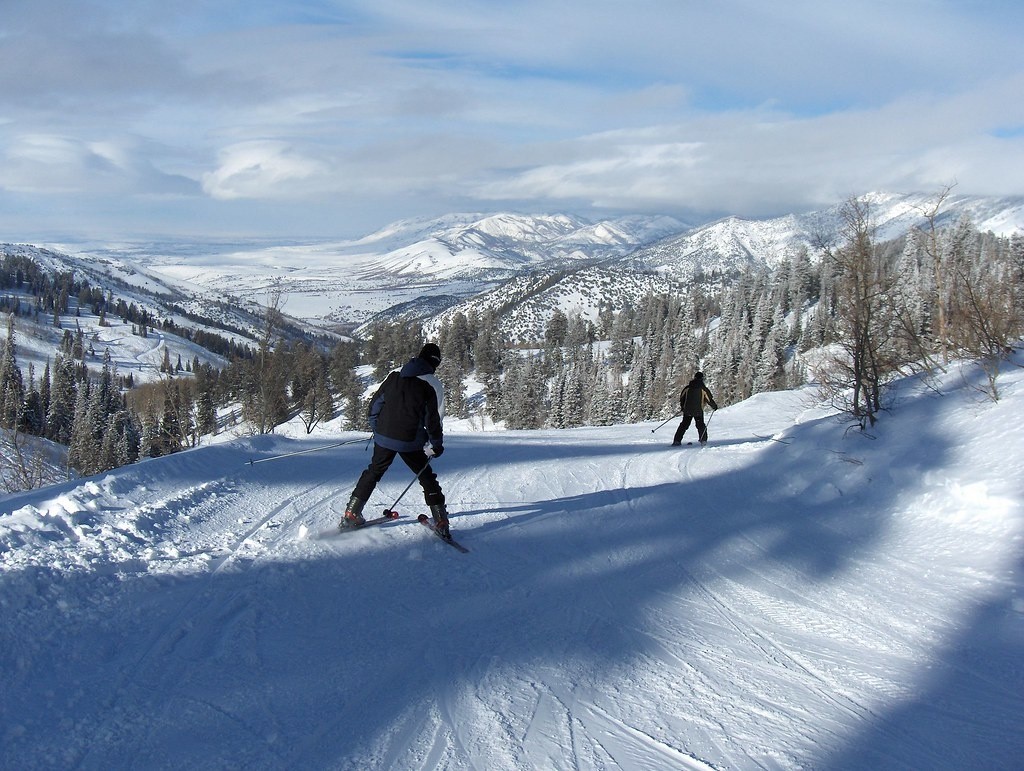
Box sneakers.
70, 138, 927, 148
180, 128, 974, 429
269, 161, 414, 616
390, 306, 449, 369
431, 504, 449, 537
345, 496, 365, 524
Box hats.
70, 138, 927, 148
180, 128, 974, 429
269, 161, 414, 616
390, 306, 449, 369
695, 372, 703, 378
419, 343, 441, 367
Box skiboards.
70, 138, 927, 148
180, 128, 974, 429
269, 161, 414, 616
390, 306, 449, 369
418, 512, 470, 555
307, 510, 399, 539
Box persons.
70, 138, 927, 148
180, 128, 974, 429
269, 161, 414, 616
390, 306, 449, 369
674, 372, 717, 446
345, 344, 450, 537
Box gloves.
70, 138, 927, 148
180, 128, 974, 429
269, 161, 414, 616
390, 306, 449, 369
431, 445, 444, 458
713, 405, 718, 411
